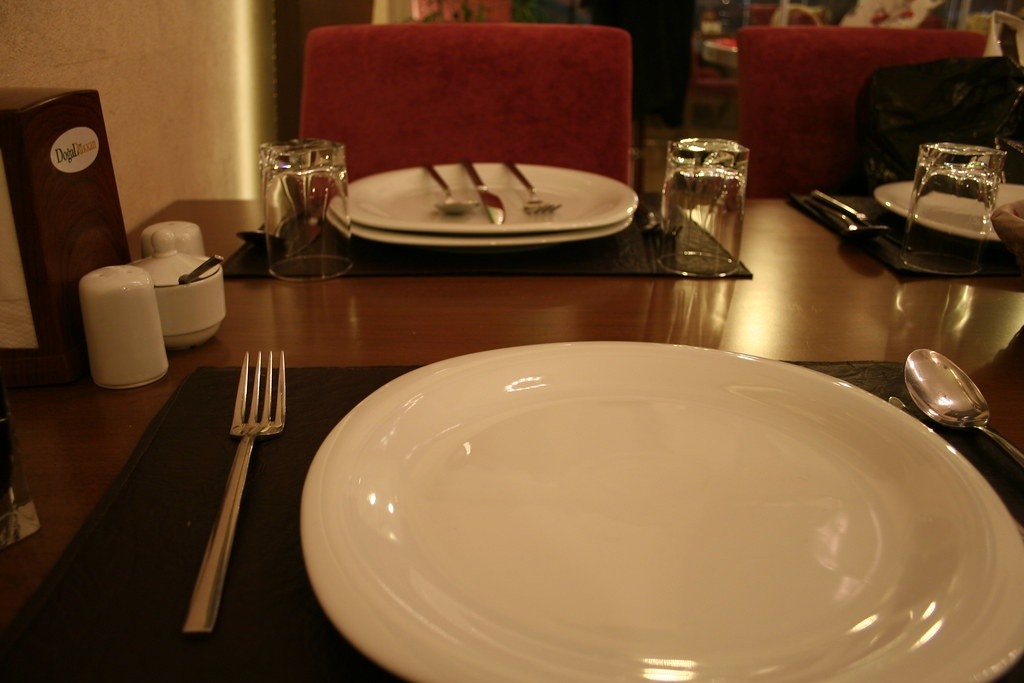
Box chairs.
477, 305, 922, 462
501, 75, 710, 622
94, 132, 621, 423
734, 27, 989, 199
684, 31, 737, 138
297, 21, 632, 206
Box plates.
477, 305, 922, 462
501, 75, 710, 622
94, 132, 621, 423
878, 180, 1024, 242
301, 341, 1024, 683
327, 161, 638, 247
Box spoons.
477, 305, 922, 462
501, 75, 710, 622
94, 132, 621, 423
417, 158, 480, 214
802, 197, 887, 235
904, 350, 1024, 468
180, 350, 287, 633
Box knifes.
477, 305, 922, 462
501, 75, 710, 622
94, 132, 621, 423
457, 155, 505, 226
810, 188, 913, 252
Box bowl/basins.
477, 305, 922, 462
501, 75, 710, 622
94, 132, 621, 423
127, 230, 226, 348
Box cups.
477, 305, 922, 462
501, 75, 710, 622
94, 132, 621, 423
662, 137, 752, 280
899, 142, 1005, 277
256, 138, 352, 280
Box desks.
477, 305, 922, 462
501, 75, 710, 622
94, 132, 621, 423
0, 197, 1024, 683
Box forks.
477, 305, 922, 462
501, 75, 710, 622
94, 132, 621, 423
502, 156, 562, 215
